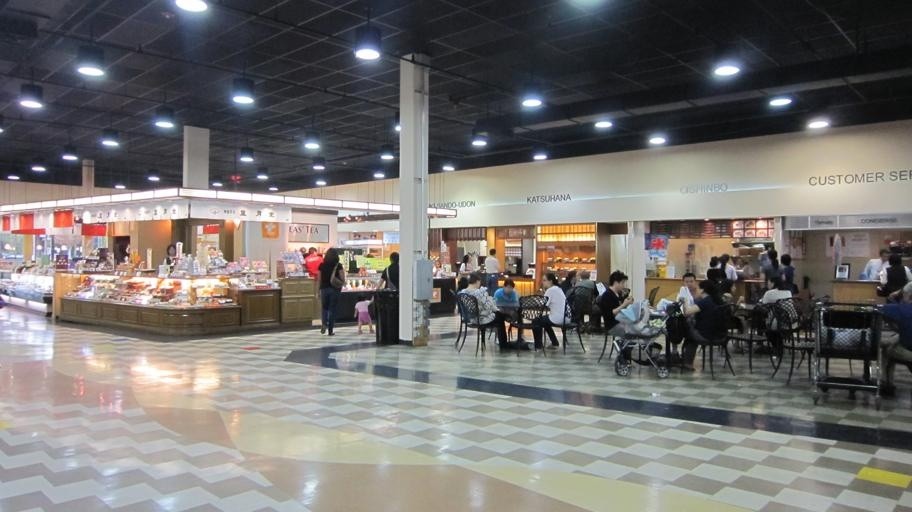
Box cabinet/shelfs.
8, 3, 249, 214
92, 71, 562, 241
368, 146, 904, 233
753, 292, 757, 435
229, 278, 282, 328
278, 276, 319, 325
1, 275, 54, 317
340, 278, 386, 325
60, 272, 240, 335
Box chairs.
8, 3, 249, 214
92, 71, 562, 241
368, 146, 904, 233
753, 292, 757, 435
573, 286, 595, 334
767, 303, 816, 386
649, 286, 660, 304
543, 295, 587, 356
766, 297, 803, 357
594, 300, 642, 364
511, 294, 549, 356
448, 289, 485, 350
681, 302, 737, 380
724, 302, 777, 373
458, 292, 504, 356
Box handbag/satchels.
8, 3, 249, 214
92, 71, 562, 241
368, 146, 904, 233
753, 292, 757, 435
386, 266, 396, 290
670, 303, 691, 338
330, 263, 344, 289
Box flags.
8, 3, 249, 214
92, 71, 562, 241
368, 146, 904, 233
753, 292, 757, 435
648, 234, 669, 259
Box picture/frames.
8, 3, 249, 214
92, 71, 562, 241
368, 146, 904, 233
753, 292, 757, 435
834, 263, 850, 279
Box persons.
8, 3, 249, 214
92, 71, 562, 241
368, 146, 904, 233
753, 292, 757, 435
599, 270, 634, 354
678, 279, 731, 372
353, 293, 375, 334
676, 272, 699, 327
717, 253, 738, 281
432, 260, 446, 279
730, 255, 755, 280
303, 247, 324, 279
484, 249, 501, 298
754, 274, 799, 357
722, 281, 747, 353
780, 253, 796, 297
560, 270, 578, 331
376, 251, 399, 290
879, 252, 912, 304
456, 271, 512, 350
493, 277, 526, 342
574, 269, 599, 335
864, 248, 890, 280
878, 280, 912, 399
707, 256, 727, 286
760, 248, 783, 291
457, 254, 475, 314
316, 247, 346, 336
528, 272, 572, 352
162, 244, 179, 274
758, 243, 772, 281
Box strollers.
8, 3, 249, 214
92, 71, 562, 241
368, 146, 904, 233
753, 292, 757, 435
607, 296, 686, 380
